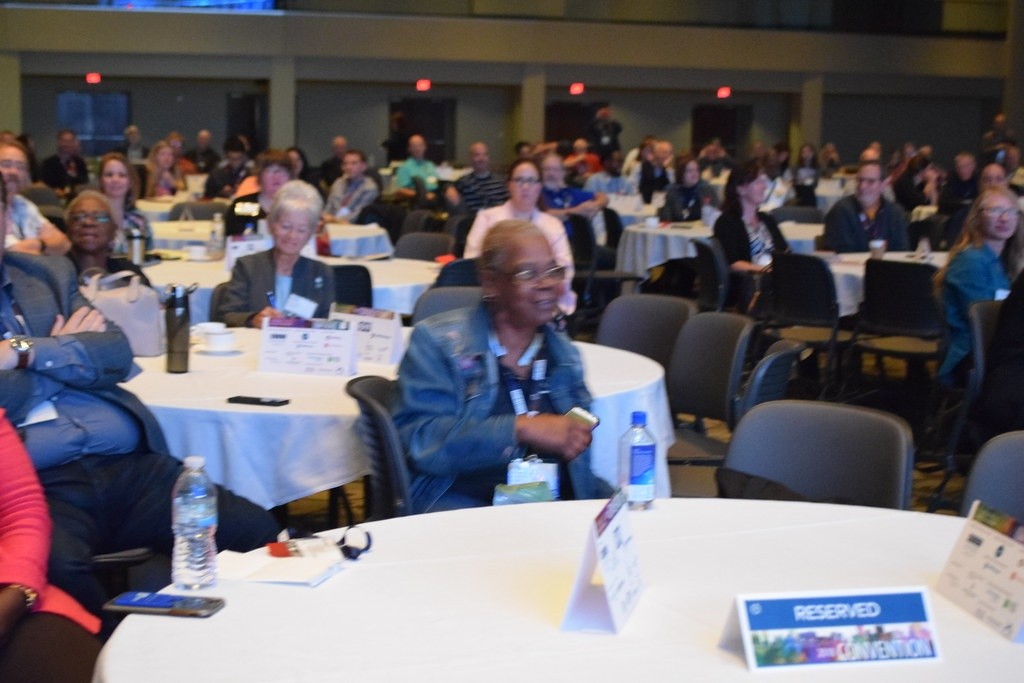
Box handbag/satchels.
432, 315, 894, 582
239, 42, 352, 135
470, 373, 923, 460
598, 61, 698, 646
78, 268, 161, 358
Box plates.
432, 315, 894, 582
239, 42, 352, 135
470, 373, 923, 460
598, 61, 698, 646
191, 344, 240, 354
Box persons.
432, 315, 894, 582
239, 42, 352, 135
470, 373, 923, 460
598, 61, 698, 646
981, 114, 1015, 152
585, 107, 623, 160
0, 406, 103, 683
0, 124, 1024, 602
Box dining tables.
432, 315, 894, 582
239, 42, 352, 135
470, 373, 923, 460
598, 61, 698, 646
618, 219, 833, 290
826, 248, 952, 315
134, 196, 254, 220
601, 192, 667, 217
818, 177, 857, 196
117, 315, 674, 527
136, 255, 455, 326
147, 215, 395, 257
87, 505, 1023, 682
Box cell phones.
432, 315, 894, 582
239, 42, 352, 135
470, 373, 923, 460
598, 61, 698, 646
563, 407, 600, 431
228, 396, 289, 406
102, 591, 224, 619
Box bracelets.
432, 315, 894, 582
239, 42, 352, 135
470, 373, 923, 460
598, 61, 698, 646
10, 585, 39, 609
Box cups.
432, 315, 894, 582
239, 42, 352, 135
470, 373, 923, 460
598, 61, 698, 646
645, 216, 659, 228
204, 330, 237, 351
127, 234, 147, 266
189, 321, 226, 338
870, 240, 886, 259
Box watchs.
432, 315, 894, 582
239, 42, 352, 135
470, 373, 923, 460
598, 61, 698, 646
10, 335, 32, 369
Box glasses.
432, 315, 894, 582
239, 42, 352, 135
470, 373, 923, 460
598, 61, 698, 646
487, 266, 565, 286
67, 214, 110, 223
978, 207, 1020, 220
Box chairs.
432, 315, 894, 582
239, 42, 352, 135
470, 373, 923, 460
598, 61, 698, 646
20, 181, 1024, 579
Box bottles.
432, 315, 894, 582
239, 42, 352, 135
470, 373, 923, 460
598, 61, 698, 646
170, 456, 219, 593
617, 411, 657, 510
164, 282, 199, 373
207, 212, 224, 258
